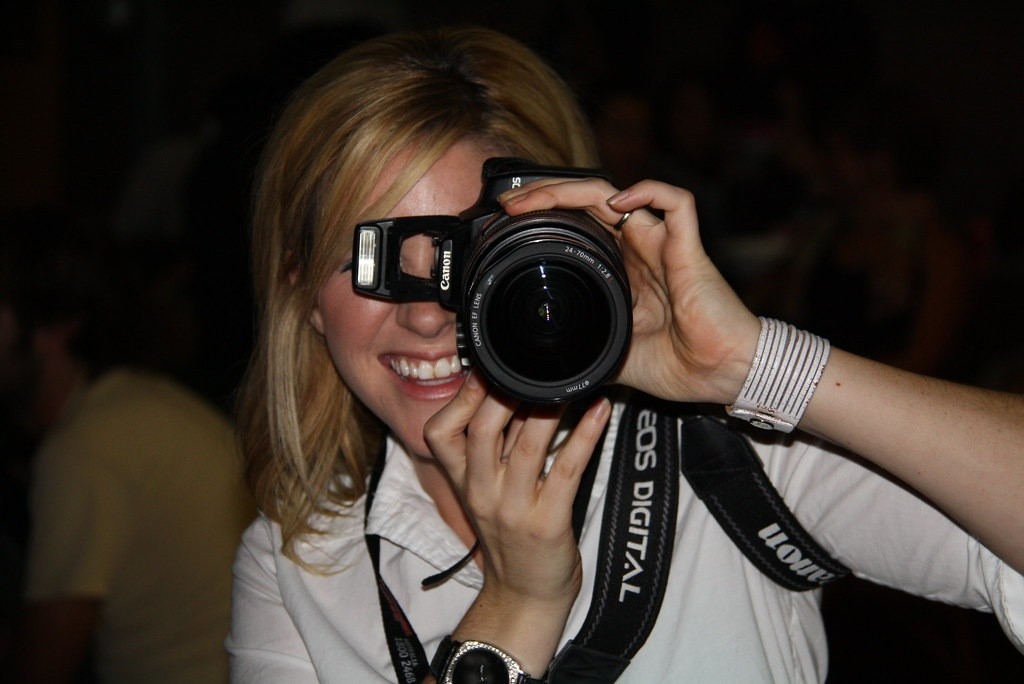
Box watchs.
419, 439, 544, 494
427, 633, 543, 684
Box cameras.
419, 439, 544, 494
349, 157, 632, 409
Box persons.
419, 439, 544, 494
1, 248, 254, 684
201, 23, 1024, 684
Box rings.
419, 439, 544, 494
613, 209, 635, 230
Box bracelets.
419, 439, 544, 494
724, 315, 832, 435
